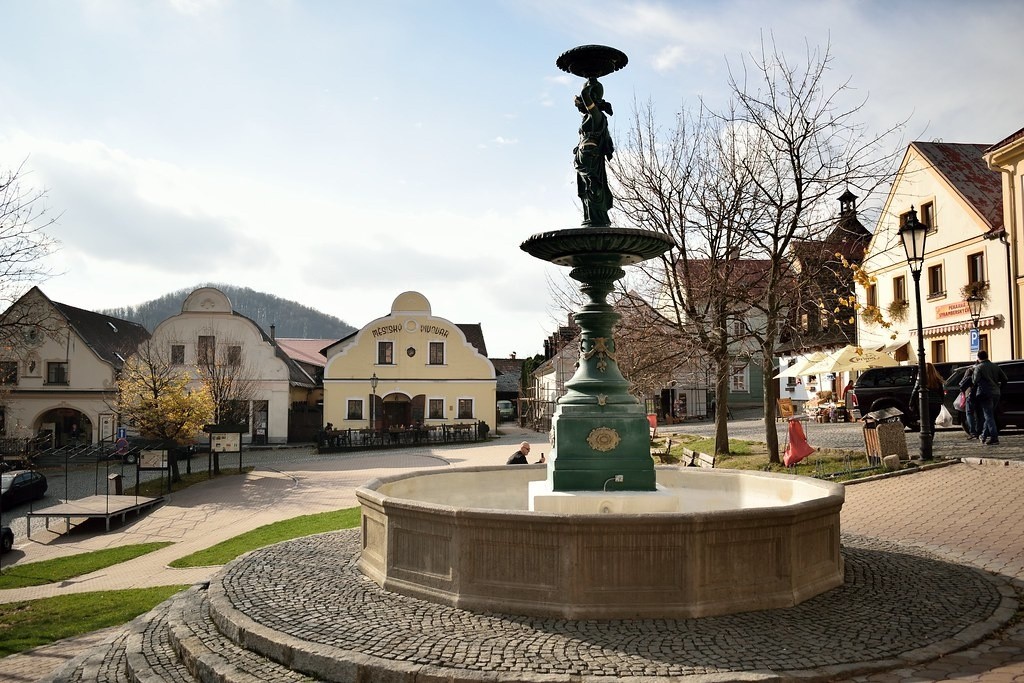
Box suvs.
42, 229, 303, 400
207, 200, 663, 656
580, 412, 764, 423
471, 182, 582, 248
850, 358, 1024, 436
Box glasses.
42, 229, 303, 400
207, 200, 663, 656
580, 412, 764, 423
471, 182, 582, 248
523, 446, 530, 452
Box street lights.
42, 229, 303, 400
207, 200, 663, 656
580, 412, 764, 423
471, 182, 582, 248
369, 372, 379, 438
965, 289, 983, 362
895, 204, 934, 461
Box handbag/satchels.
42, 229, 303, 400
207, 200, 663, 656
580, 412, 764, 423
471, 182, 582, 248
935, 404, 953, 427
991, 382, 1000, 395
953, 392, 964, 411
965, 387, 972, 397
960, 392, 966, 408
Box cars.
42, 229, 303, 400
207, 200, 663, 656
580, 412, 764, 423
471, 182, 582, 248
1, 470, 48, 512
124, 434, 197, 463
0, 526, 14, 553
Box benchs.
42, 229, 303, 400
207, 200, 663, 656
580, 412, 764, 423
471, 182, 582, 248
649, 427, 715, 468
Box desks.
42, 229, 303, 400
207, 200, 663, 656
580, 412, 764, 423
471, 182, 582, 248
808, 407, 845, 422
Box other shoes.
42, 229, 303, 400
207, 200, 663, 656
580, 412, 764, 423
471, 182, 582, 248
986, 439, 1000, 445
979, 435, 986, 444
966, 434, 977, 440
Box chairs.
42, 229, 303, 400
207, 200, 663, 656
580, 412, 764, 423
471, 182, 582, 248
318, 422, 472, 449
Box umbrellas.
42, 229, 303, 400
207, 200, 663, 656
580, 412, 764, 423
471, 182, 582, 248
772, 345, 900, 399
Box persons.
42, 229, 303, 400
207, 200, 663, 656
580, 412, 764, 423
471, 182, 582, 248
909, 362, 945, 441
574, 79, 613, 226
324, 423, 335, 446
972, 350, 1008, 444
507, 442, 545, 465
711, 395, 715, 423
68, 424, 81, 449
411, 420, 428, 442
960, 366, 984, 439
842, 380, 856, 406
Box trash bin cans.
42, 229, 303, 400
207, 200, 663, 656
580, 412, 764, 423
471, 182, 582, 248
860, 407, 910, 466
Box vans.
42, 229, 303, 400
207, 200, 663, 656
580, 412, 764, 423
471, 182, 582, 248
497, 400, 516, 420
